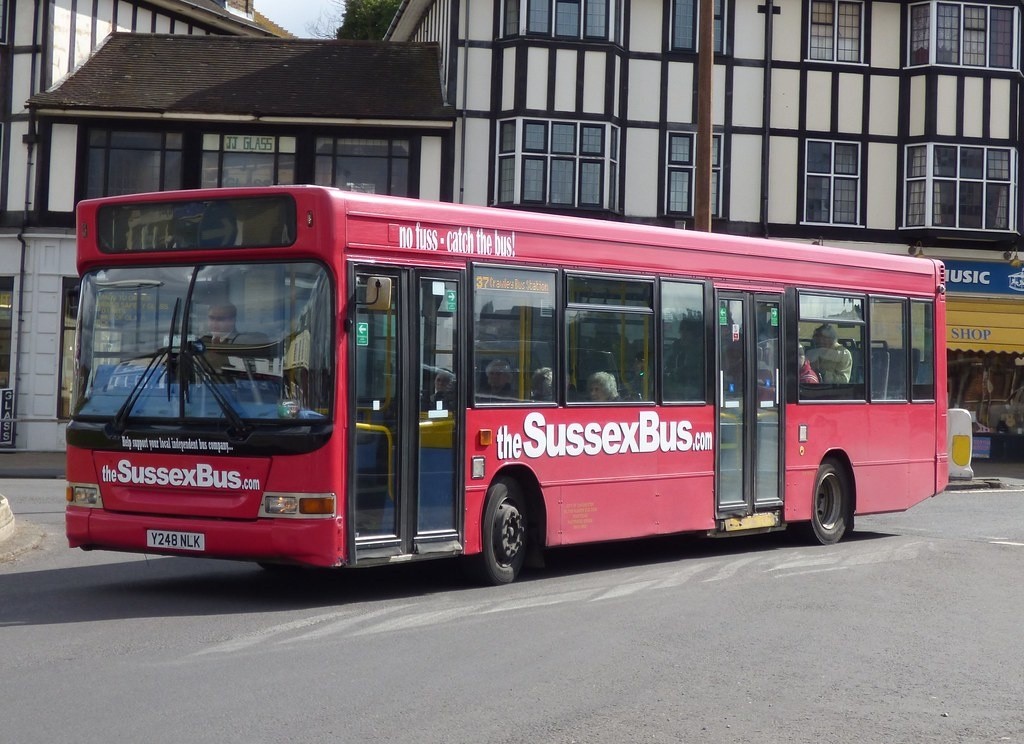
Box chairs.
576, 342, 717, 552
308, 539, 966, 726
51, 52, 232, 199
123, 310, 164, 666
801, 336, 920, 397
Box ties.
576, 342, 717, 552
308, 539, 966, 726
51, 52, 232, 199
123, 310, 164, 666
210, 336, 220, 356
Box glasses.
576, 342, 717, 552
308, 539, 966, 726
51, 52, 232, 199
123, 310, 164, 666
208, 315, 232, 322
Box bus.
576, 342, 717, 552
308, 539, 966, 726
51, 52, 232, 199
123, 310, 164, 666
59, 184, 951, 590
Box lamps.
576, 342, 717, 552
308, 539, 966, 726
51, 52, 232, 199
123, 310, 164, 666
910, 241, 926, 258
1004, 245, 1021, 267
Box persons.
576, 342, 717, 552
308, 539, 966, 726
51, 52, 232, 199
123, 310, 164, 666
668, 317, 852, 402
429, 351, 673, 412
195, 300, 248, 373
